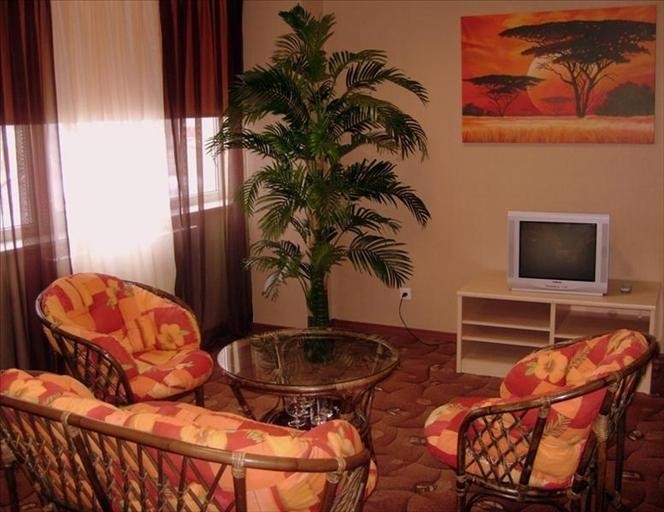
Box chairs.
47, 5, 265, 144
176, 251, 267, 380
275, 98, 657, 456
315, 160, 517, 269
35, 272, 214, 406
422, 326, 659, 512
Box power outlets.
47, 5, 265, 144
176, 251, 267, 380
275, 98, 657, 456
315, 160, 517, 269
399, 289, 412, 300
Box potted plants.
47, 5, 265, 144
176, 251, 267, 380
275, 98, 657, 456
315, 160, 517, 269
204, 2, 431, 364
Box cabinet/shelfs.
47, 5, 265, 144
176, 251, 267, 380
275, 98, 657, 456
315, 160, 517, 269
456, 270, 661, 395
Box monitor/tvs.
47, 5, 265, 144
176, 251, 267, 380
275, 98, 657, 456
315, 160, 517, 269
507, 210, 610, 296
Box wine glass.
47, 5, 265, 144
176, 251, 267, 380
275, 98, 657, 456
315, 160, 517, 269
283, 394, 316, 429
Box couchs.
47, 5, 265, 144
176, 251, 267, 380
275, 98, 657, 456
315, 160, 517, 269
0, 367, 379, 512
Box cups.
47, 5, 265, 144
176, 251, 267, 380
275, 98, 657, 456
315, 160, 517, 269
310, 397, 334, 426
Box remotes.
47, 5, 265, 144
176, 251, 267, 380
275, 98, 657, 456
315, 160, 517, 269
620, 283, 632, 293
